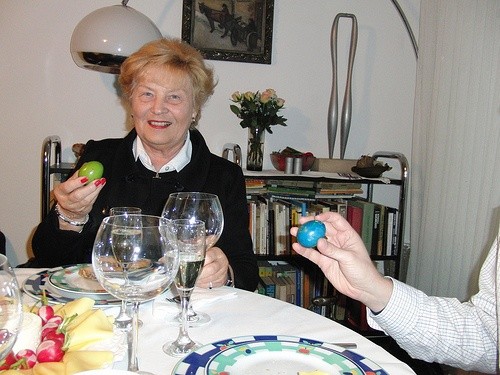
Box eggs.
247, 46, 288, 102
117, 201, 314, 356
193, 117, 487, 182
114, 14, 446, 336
296, 219, 326, 248
78, 161, 104, 186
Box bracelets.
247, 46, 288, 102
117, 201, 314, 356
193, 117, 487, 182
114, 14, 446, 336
54, 203, 89, 226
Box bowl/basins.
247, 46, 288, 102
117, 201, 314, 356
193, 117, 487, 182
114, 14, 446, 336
351, 166, 392, 177
269, 154, 316, 171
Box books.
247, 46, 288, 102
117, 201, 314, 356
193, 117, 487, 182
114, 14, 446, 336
255, 261, 367, 331
249, 200, 399, 256
245, 178, 364, 201
373, 260, 396, 278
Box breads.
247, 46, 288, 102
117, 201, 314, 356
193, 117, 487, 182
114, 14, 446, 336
97, 255, 150, 272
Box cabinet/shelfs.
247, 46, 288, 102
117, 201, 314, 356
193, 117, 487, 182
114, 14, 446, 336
223, 152, 408, 358
41, 134, 77, 218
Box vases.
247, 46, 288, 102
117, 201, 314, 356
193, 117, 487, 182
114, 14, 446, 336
245, 126, 266, 172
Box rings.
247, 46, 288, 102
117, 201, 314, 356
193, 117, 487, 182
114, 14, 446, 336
209, 282, 212, 289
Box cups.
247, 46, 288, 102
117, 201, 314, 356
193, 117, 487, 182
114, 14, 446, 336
0, 254, 23, 363
284, 157, 303, 175
4, 312, 43, 358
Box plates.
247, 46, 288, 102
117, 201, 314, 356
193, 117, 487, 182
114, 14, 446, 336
21, 263, 169, 308
204, 340, 366, 375
48, 263, 111, 295
170, 335, 391, 375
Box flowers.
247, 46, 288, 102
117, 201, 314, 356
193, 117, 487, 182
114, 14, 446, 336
229, 88, 289, 166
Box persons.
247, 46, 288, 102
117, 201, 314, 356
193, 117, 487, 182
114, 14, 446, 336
15, 36, 259, 293
290, 211, 500, 374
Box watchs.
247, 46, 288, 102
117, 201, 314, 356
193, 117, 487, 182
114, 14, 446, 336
224, 267, 232, 286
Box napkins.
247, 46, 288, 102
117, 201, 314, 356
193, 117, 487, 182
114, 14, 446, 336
148, 284, 236, 319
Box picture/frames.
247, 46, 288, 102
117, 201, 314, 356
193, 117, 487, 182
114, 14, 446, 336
181, 0, 275, 66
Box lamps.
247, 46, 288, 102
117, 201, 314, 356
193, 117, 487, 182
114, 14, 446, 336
69, 0, 164, 76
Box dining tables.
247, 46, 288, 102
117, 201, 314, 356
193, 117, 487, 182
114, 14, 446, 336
0, 265, 416, 375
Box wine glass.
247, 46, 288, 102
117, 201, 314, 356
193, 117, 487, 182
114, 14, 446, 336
109, 207, 143, 331
92, 214, 179, 375
158, 192, 224, 329
162, 218, 204, 358
